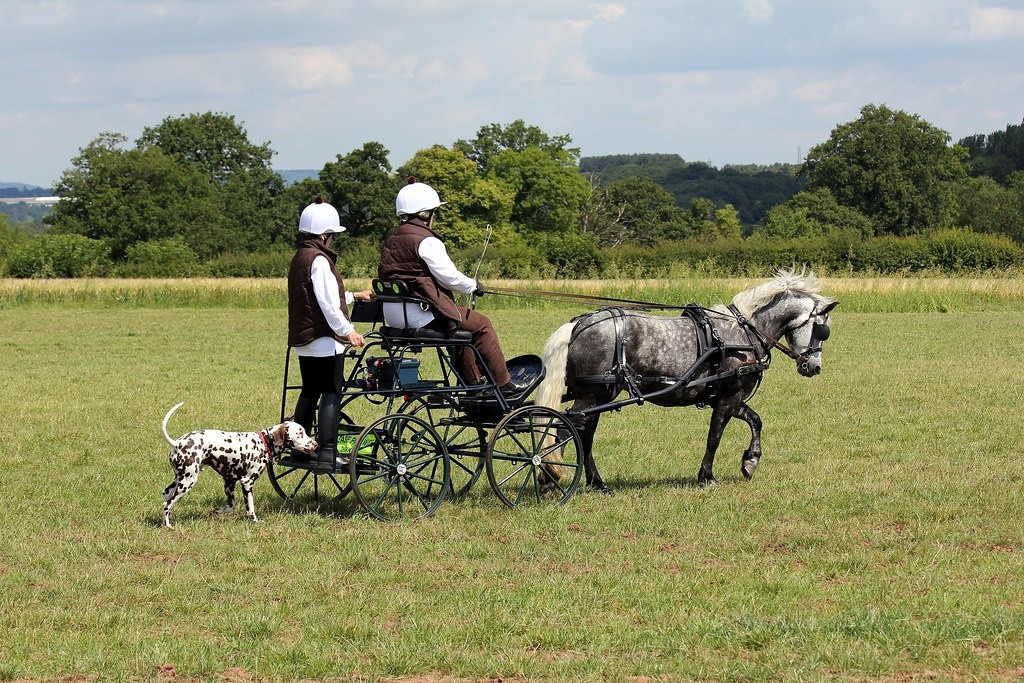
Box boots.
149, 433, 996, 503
289, 397, 318, 462
319, 400, 358, 469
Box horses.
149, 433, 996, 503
535, 258, 839, 500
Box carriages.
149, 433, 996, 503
266, 264, 840, 523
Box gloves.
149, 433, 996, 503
473, 282, 484, 297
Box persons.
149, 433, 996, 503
286, 197, 375, 468
380, 177, 537, 396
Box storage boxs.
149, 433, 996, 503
365, 356, 421, 387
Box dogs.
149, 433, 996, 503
162, 402, 319, 530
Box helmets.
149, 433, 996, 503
298, 197, 347, 235
396, 176, 447, 216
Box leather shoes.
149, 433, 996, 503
473, 375, 488, 395
500, 377, 537, 399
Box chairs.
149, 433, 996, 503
373, 280, 514, 408
